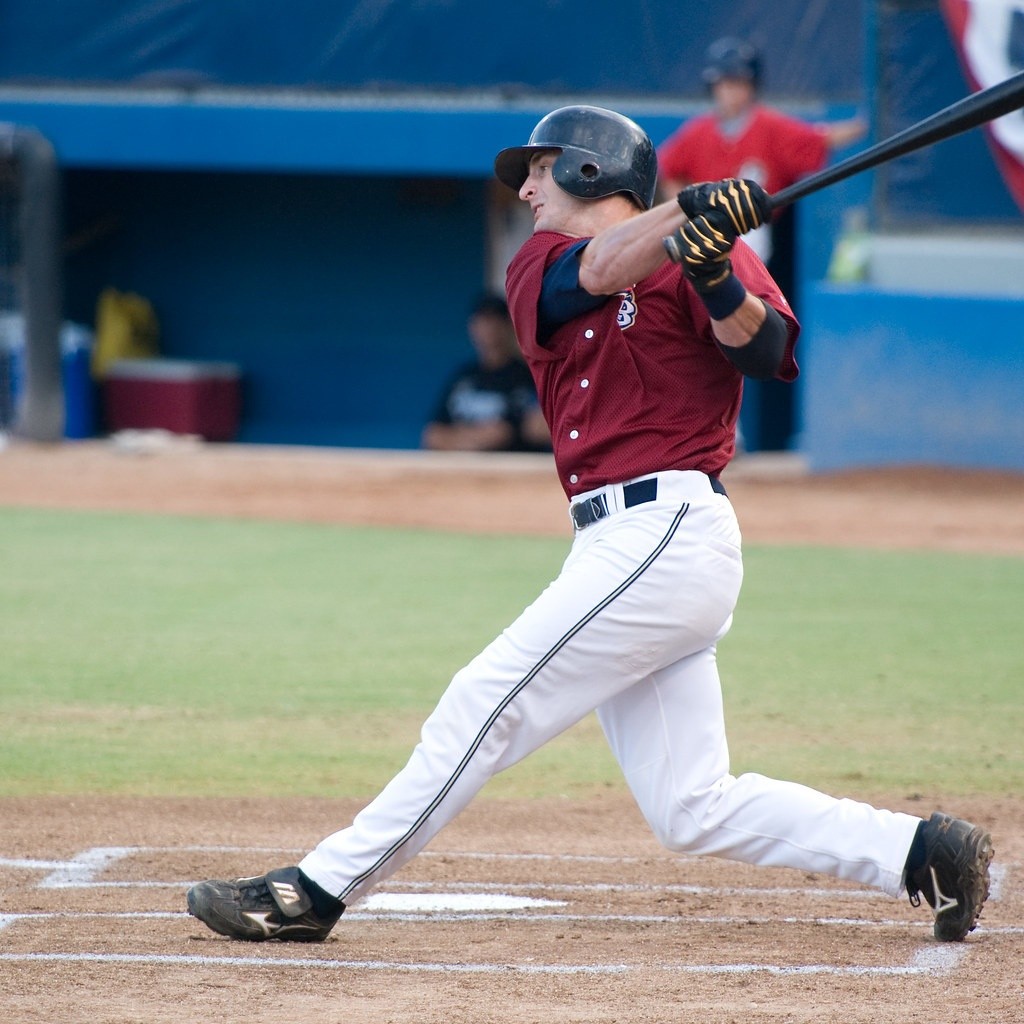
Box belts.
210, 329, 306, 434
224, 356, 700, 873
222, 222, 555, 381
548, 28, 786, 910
570, 476, 729, 529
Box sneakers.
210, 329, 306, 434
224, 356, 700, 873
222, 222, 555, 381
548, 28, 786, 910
186, 866, 346, 943
906, 812, 995, 942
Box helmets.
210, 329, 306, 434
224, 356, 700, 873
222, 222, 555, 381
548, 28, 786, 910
700, 38, 765, 88
494, 105, 658, 209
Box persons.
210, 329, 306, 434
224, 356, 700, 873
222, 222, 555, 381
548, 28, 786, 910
189, 108, 994, 945
432, 38, 861, 453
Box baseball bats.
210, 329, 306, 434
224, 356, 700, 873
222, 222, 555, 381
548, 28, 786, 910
661, 69, 1024, 261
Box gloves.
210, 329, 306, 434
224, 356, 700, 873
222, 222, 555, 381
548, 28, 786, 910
674, 210, 746, 322
677, 177, 773, 236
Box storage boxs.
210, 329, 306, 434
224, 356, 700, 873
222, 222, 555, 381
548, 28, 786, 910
100, 357, 247, 441
0, 311, 95, 441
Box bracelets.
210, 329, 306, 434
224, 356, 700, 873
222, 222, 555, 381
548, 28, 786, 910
696, 270, 747, 323
